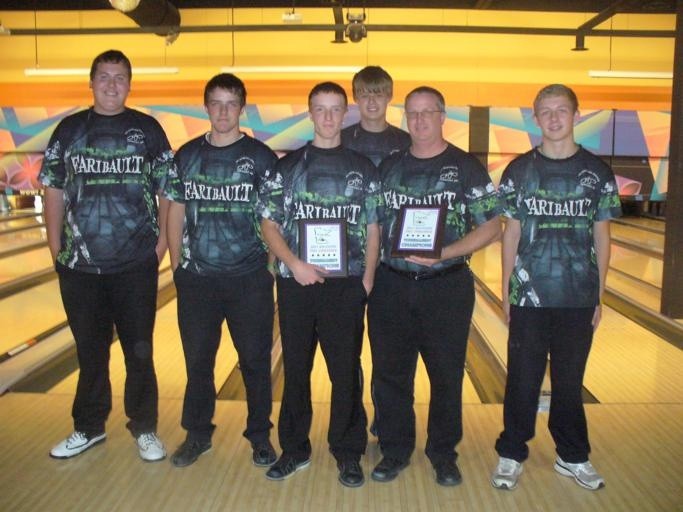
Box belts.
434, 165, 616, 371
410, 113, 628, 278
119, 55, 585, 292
379, 262, 463, 283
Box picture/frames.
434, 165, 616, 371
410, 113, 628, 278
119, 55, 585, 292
297, 216, 351, 281
387, 201, 447, 262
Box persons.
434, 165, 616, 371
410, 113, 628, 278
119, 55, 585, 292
370, 86, 504, 486
36, 49, 174, 461
494, 83, 624, 490
254, 82, 386, 488
340, 66, 411, 168
161, 73, 279, 466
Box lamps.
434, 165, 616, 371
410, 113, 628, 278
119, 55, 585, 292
340, 2, 370, 46
108, 0, 179, 38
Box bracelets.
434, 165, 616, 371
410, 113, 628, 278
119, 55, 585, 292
266, 264, 274, 269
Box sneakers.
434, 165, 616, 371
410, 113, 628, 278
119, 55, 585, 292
172, 438, 211, 466
251, 442, 278, 466
372, 457, 410, 481
491, 458, 523, 488
553, 456, 605, 490
328, 447, 365, 487
429, 454, 461, 485
48, 432, 106, 460
267, 454, 311, 481
136, 435, 166, 461
